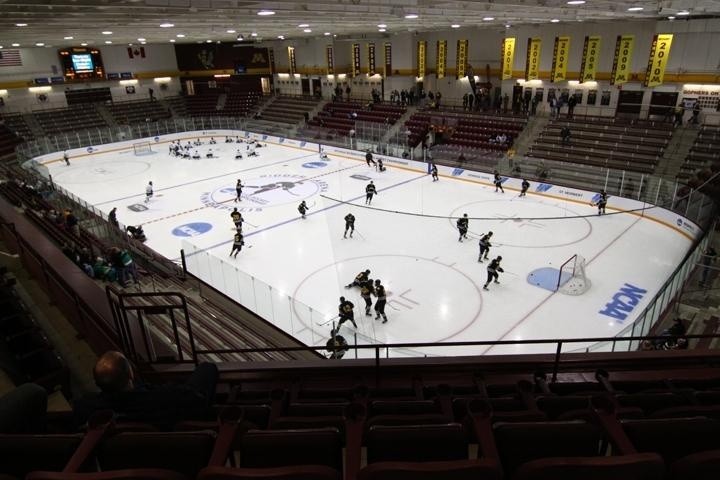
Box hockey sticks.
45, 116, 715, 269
244, 243, 253, 248
243, 222, 260, 228
467, 231, 484, 236
315, 316, 338, 327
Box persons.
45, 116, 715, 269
699, 247, 718, 284
664, 100, 700, 125
167, 134, 263, 160
299, 201, 308, 216
458, 153, 465, 162
463, 91, 539, 116
484, 256, 504, 290
365, 181, 377, 204
304, 112, 310, 128
550, 93, 577, 119
314, 85, 442, 172
431, 164, 438, 181
343, 213, 355, 238
596, 190, 607, 214
488, 131, 546, 197
255, 112, 262, 120
72, 350, 220, 432
63, 154, 71, 166
561, 127, 571, 143
0, 382, 48, 433
228, 178, 246, 258
478, 232, 493, 263
457, 213, 469, 242
11, 175, 154, 289
659, 319, 688, 351
326, 269, 388, 359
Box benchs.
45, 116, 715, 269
253, 85, 718, 181
635, 314, 718, 349
0, 87, 270, 154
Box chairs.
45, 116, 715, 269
0, 349, 719, 479
1, 155, 325, 364
